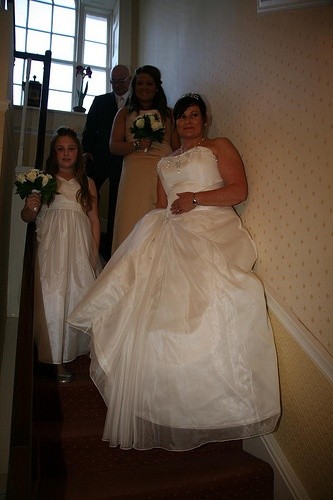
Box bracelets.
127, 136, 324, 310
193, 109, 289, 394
192, 193, 197, 207
133, 140, 140, 151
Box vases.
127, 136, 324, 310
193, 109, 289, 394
73, 81, 88, 113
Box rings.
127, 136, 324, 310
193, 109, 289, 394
177, 210, 180, 212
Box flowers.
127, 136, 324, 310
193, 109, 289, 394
128, 113, 166, 143
15, 169, 61, 208
75, 65, 92, 93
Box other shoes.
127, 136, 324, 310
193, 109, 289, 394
53, 364, 73, 384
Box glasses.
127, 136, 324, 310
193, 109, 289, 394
110, 76, 130, 84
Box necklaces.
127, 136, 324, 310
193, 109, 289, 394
180, 137, 206, 154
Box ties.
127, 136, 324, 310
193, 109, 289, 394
117, 96, 123, 110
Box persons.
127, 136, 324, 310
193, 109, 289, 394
21, 127, 100, 385
109, 65, 181, 256
81, 65, 132, 263
65, 93, 281, 450
